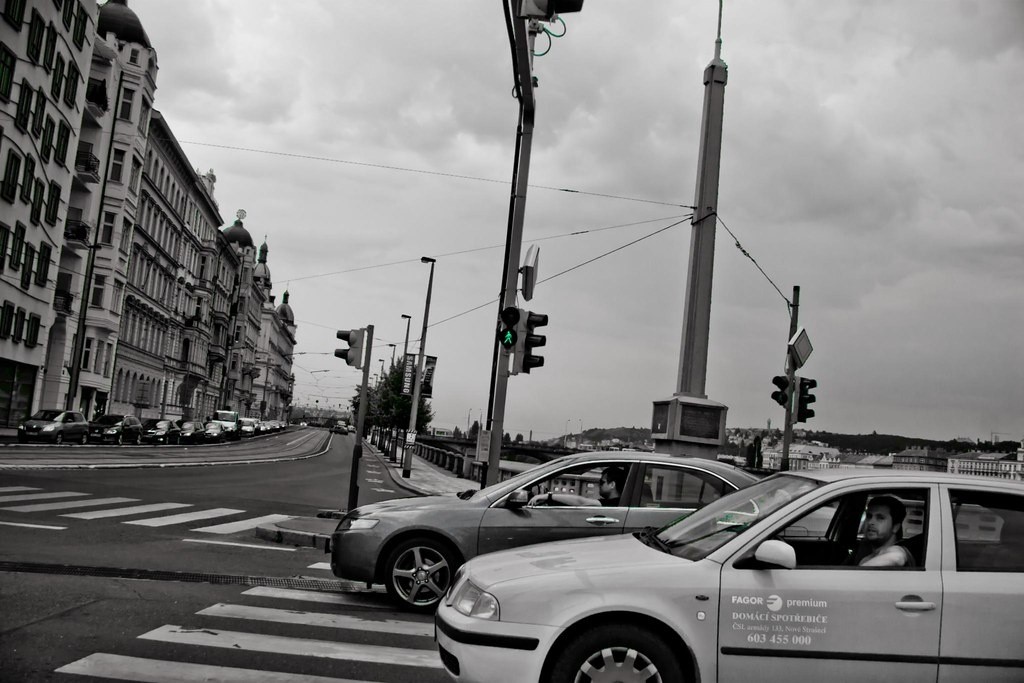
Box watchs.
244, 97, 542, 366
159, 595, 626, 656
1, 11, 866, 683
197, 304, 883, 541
547, 491, 553, 500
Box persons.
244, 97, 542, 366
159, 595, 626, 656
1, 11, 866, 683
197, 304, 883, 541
527, 467, 626, 508
858, 496, 913, 567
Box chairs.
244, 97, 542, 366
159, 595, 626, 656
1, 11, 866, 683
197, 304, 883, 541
971, 517, 1024, 571
640, 483, 654, 507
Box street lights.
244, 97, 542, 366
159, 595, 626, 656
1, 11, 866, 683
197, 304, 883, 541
798, 377, 817, 423
402, 257, 437, 478
369, 358, 385, 450
771, 376, 789, 405
390, 314, 411, 460
523, 311, 548, 374
384, 343, 396, 456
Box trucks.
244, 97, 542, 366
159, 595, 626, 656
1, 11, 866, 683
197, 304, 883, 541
207, 410, 242, 441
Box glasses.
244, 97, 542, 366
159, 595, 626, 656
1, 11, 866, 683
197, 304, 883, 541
599, 478, 607, 486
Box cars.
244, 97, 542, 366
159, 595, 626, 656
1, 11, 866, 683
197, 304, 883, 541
203, 422, 226, 443
18, 409, 89, 445
89, 414, 143, 445
238, 418, 286, 437
330, 450, 866, 613
141, 419, 182, 445
175, 420, 206, 444
329, 421, 356, 435
435, 468, 1024, 683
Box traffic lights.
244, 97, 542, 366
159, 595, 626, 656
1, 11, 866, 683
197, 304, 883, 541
334, 329, 364, 366
500, 306, 520, 349
